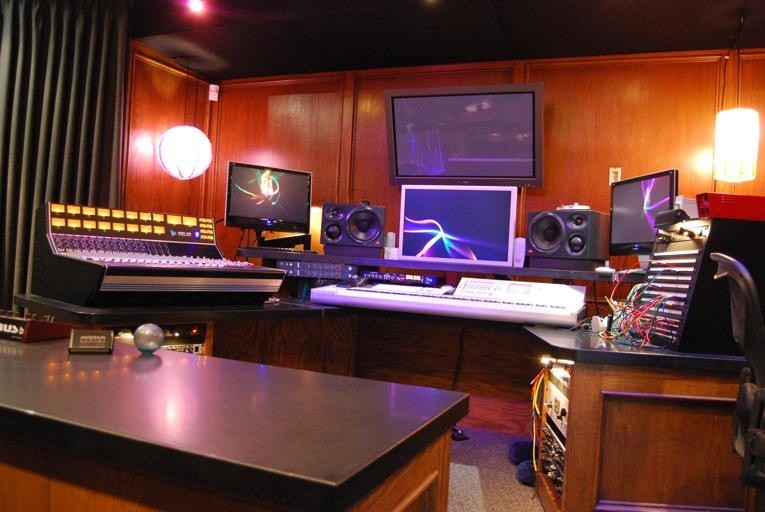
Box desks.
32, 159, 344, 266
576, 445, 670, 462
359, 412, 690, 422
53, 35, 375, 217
0, 330, 467, 512
525, 324, 750, 512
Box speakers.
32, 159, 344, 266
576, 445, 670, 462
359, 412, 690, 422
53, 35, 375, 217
320, 202, 385, 249
525, 209, 604, 261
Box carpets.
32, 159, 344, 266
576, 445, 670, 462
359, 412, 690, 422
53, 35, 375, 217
448, 427, 542, 512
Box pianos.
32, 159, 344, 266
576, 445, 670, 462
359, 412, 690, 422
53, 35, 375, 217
311, 284, 588, 327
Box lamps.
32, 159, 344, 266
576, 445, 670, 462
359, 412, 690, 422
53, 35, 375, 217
157, 55, 213, 181
708, 8, 759, 183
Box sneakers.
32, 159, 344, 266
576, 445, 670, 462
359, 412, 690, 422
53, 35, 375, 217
516, 464, 536, 486
509, 442, 536, 463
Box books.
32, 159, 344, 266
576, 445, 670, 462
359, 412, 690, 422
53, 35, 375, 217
69, 329, 114, 353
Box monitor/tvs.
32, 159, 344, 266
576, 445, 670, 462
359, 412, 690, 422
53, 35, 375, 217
399, 183, 518, 266
383, 81, 545, 191
224, 161, 312, 234
608, 168, 679, 256
